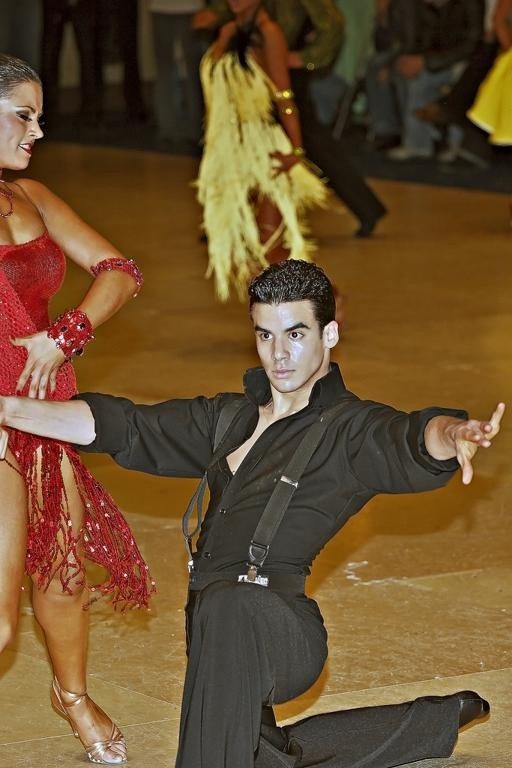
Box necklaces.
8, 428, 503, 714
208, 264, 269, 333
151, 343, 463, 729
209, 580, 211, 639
0, 179, 16, 218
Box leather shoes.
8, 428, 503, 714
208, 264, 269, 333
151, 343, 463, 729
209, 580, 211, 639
453, 688, 489, 727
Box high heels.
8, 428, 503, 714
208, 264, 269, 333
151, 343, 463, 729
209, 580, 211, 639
49, 677, 128, 766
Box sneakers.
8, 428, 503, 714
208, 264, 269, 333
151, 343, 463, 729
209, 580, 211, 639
355, 205, 387, 239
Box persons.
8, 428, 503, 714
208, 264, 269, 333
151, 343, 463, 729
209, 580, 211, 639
191, 2, 388, 298
0, 2, 205, 149
0, 55, 142, 764
311, 2, 511, 167
0, 258, 504, 768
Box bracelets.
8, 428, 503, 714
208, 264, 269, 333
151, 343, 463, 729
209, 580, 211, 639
45, 308, 95, 361
